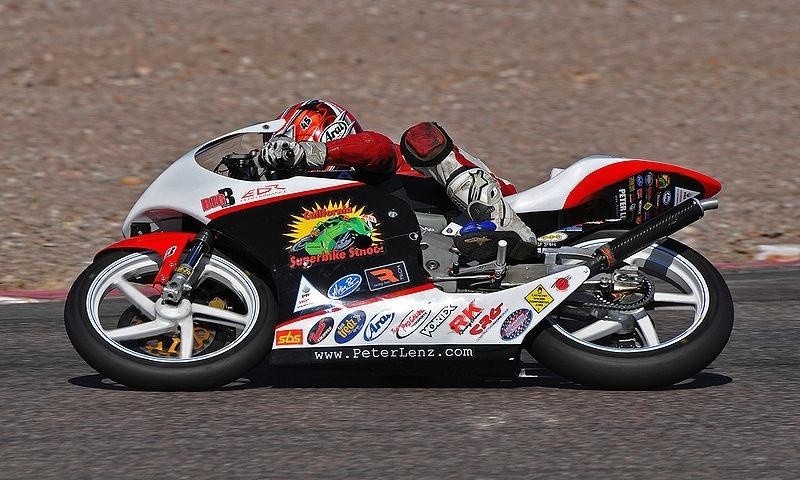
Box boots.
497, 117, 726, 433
446, 168, 538, 267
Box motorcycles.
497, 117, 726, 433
63, 116, 735, 390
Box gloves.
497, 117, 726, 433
258, 134, 328, 181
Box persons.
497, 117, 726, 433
259, 97, 537, 260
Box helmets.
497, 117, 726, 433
262, 99, 363, 174
